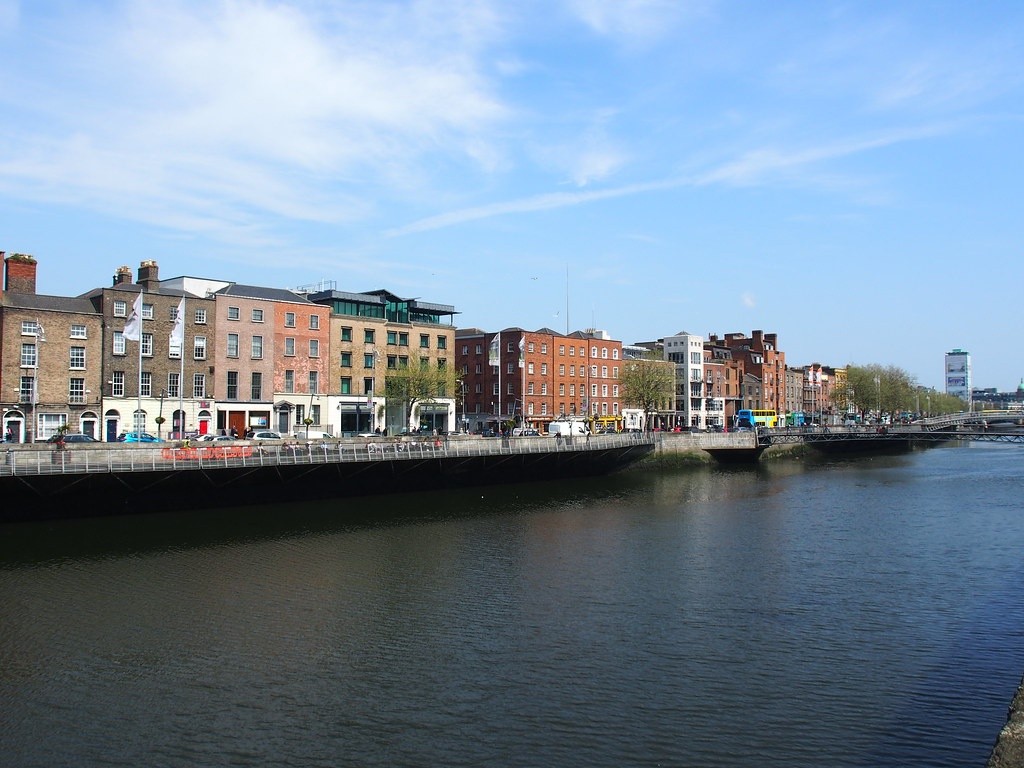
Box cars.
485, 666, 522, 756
298, 431, 332, 439
190, 434, 220, 442
245, 431, 281, 440
653, 425, 776, 434
117, 432, 165, 443
519, 430, 542, 436
598, 430, 617, 434
212, 436, 244, 441
394, 432, 420, 436
621, 428, 640, 433
45, 434, 101, 443
452, 432, 466, 436
844, 411, 915, 428
358, 433, 382, 437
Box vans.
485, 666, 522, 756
548, 422, 586, 436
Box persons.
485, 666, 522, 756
786, 422, 791, 434
222, 427, 227, 436
412, 427, 420, 434
823, 421, 831, 433
231, 425, 253, 440
458, 426, 511, 438
375, 425, 388, 437
433, 425, 443, 438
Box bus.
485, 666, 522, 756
732, 409, 778, 428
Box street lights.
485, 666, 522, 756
30, 318, 45, 444
926, 395, 931, 418
153, 388, 171, 438
371, 347, 382, 435
455, 379, 465, 414
306, 393, 319, 439
